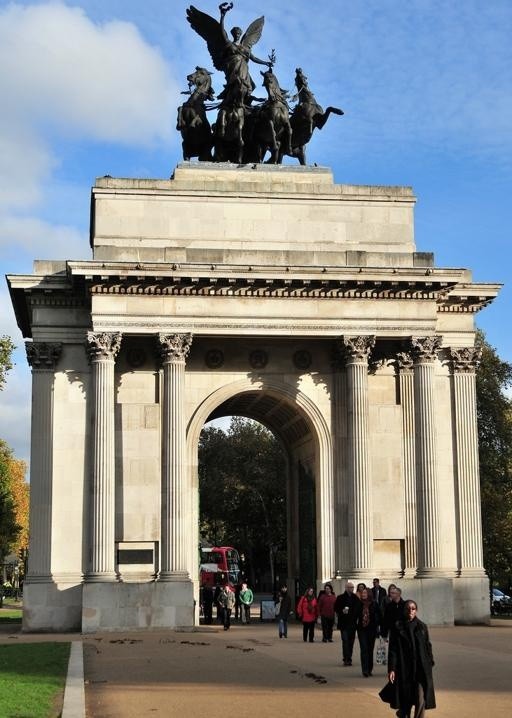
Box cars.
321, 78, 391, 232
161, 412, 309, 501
491, 588, 510, 605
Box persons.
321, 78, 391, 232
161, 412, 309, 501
200, 580, 254, 631
216, 0, 275, 92
378, 599, 436, 718
273, 583, 291, 638
295, 576, 406, 678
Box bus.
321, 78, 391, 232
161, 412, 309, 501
200, 546, 245, 591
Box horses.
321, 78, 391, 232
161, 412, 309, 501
175, 65, 345, 165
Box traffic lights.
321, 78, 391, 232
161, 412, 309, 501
18, 547, 24, 560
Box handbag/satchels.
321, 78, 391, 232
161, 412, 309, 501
275, 600, 281, 615
376, 639, 388, 665
